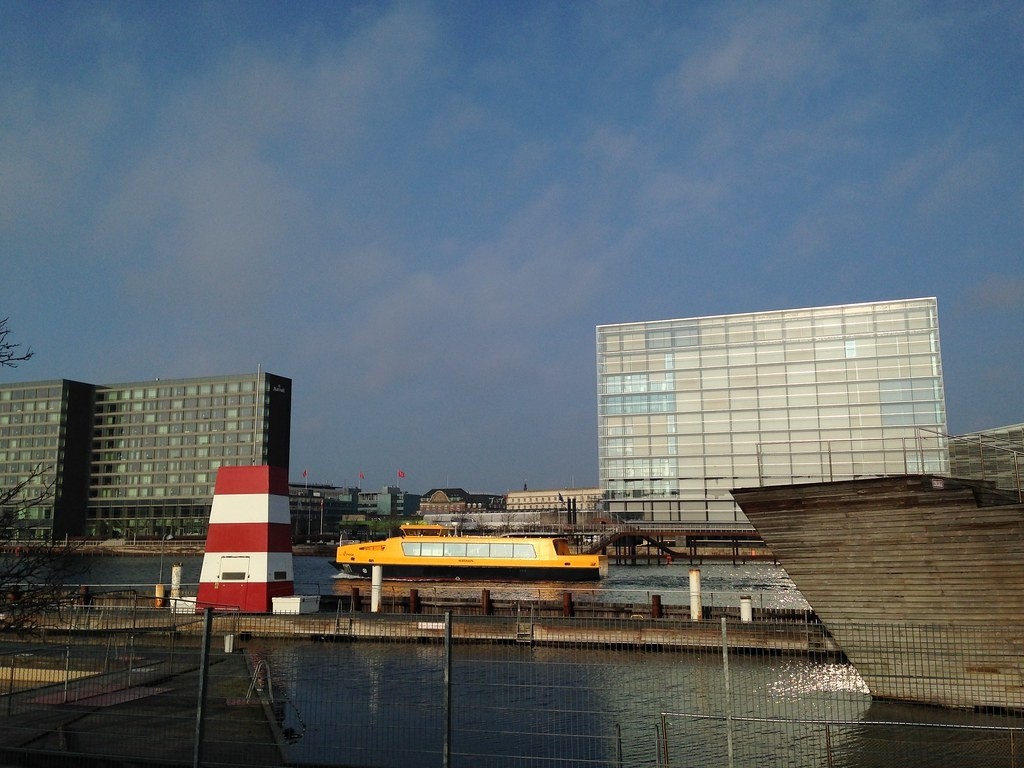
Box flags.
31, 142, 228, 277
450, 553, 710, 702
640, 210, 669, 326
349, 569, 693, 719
398, 469, 405, 479
360, 471, 364, 479
302, 469, 306, 478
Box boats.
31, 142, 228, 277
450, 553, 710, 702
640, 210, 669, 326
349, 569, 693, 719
730, 427, 1024, 717
328, 524, 609, 582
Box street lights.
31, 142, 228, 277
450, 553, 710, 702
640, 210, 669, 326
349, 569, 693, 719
158, 533, 173, 584
